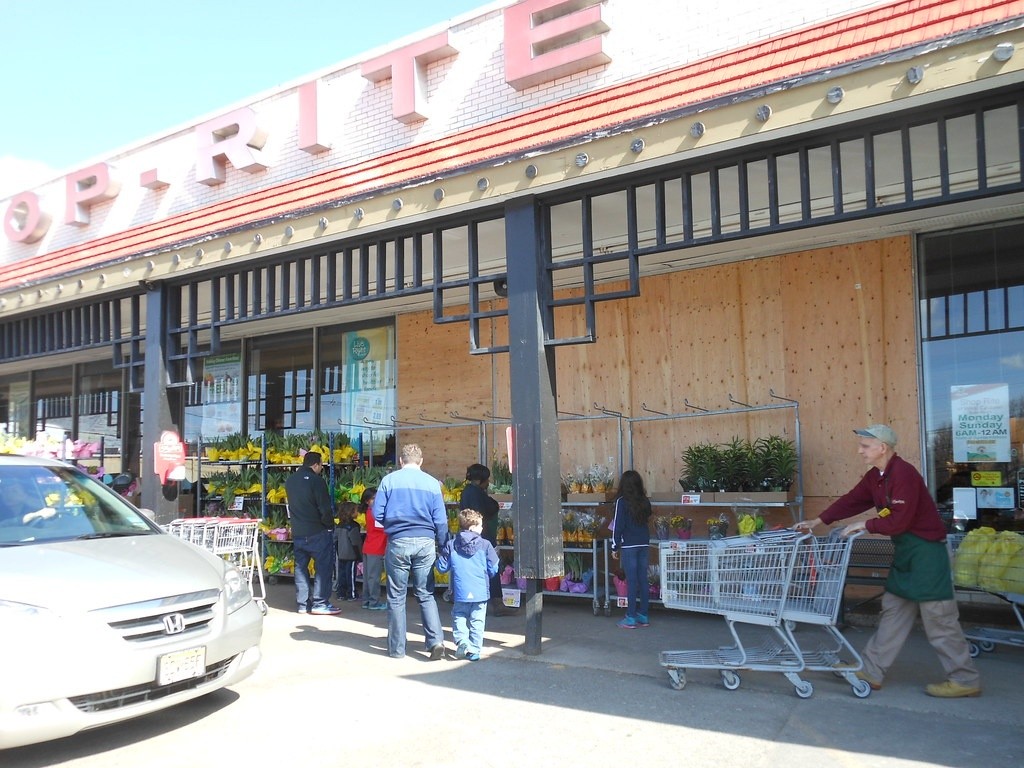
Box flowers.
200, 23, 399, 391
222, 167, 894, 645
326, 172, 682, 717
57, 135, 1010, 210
707, 516, 728, 527
670, 515, 693, 529
656, 516, 668, 526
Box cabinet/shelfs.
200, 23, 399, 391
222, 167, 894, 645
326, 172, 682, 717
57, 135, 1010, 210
64, 435, 104, 481
454, 403, 622, 507
197, 434, 266, 585
603, 538, 660, 617
337, 411, 482, 506
262, 434, 361, 583
434, 539, 602, 617
601, 390, 803, 507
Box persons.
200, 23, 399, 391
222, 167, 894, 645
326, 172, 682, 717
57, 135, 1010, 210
333, 502, 363, 602
371, 444, 449, 660
285, 452, 342, 615
459, 464, 519, 616
791, 425, 982, 698
683, 496, 690, 503
611, 470, 652, 629
0, 481, 56, 527
357, 487, 388, 610
434, 508, 499, 662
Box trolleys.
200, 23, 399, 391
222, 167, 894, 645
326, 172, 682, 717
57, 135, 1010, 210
157, 516, 269, 617
945, 532, 1024, 657
658, 518, 872, 700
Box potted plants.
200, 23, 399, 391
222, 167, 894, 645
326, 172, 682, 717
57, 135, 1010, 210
567, 467, 614, 494
196, 433, 388, 586
678, 435, 799, 492
426, 458, 512, 585
561, 511, 601, 548
565, 554, 591, 594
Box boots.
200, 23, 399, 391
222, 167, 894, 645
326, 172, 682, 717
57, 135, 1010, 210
492, 597, 519, 616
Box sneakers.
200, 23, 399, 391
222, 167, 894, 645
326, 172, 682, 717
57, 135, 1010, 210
634, 612, 649, 626
832, 662, 883, 690
311, 603, 342, 615
924, 680, 981, 698
615, 613, 637, 629
298, 610, 308, 614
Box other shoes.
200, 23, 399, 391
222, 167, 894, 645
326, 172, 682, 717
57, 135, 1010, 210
466, 651, 479, 661
348, 597, 362, 601
336, 595, 345, 601
369, 601, 387, 610
455, 640, 468, 659
430, 643, 445, 660
363, 601, 369, 608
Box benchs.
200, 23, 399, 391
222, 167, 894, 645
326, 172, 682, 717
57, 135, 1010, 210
842, 537, 898, 627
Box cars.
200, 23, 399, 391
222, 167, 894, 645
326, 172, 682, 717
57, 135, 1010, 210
0, 456, 265, 751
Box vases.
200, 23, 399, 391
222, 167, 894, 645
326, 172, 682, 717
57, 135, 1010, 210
709, 527, 728, 541
654, 526, 669, 540
677, 528, 691, 539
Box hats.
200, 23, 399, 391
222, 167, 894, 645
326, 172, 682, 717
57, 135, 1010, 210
852, 424, 897, 448
